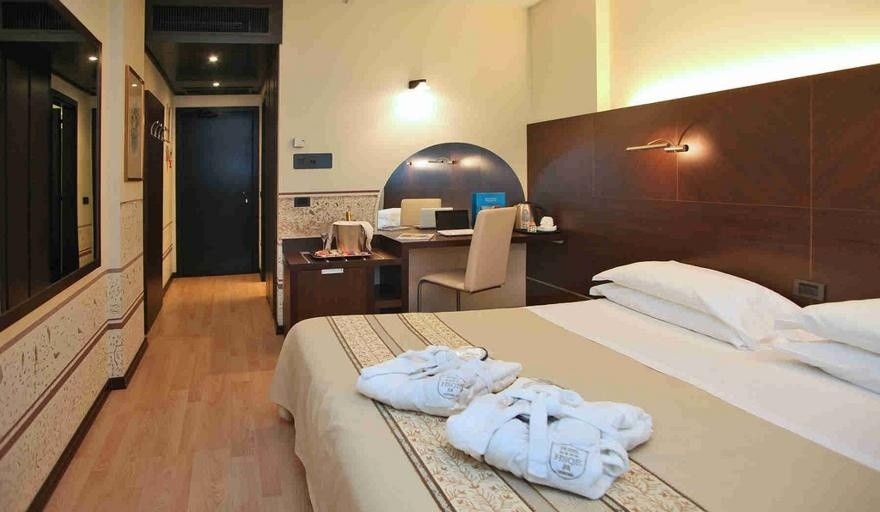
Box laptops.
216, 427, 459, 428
435, 210, 474, 237
412, 207, 453, 229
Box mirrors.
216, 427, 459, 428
375, 142, 526, 228
52, 74, 96, 286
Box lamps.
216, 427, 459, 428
409, 80, 426, 88
626, 137, 688, 152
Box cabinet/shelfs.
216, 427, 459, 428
282, 234, 401, 338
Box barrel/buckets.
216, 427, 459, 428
333, 224, 368, 251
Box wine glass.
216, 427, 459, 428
320, 229, 329, 251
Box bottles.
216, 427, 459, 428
346, 211, 351, 220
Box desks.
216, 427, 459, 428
378, 230, 564, 313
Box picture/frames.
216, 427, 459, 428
125, 64, 144, 182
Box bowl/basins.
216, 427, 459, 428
540, 216, 554, 228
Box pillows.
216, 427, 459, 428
588, 282, 745, 351
766, 340, 879, 394
592, 260, 803, 350
792, 298, 879, 354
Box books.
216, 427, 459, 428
396, 233, 435, 242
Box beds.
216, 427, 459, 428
267, 298, 879, 511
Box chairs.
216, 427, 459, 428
417, 207, 517, 312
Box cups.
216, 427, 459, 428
528, 225, 537, 233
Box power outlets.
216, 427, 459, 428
294, 197, 310, 207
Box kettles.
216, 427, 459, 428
512, 201, 545, 232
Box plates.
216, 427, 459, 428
537, 225, 557, 232
